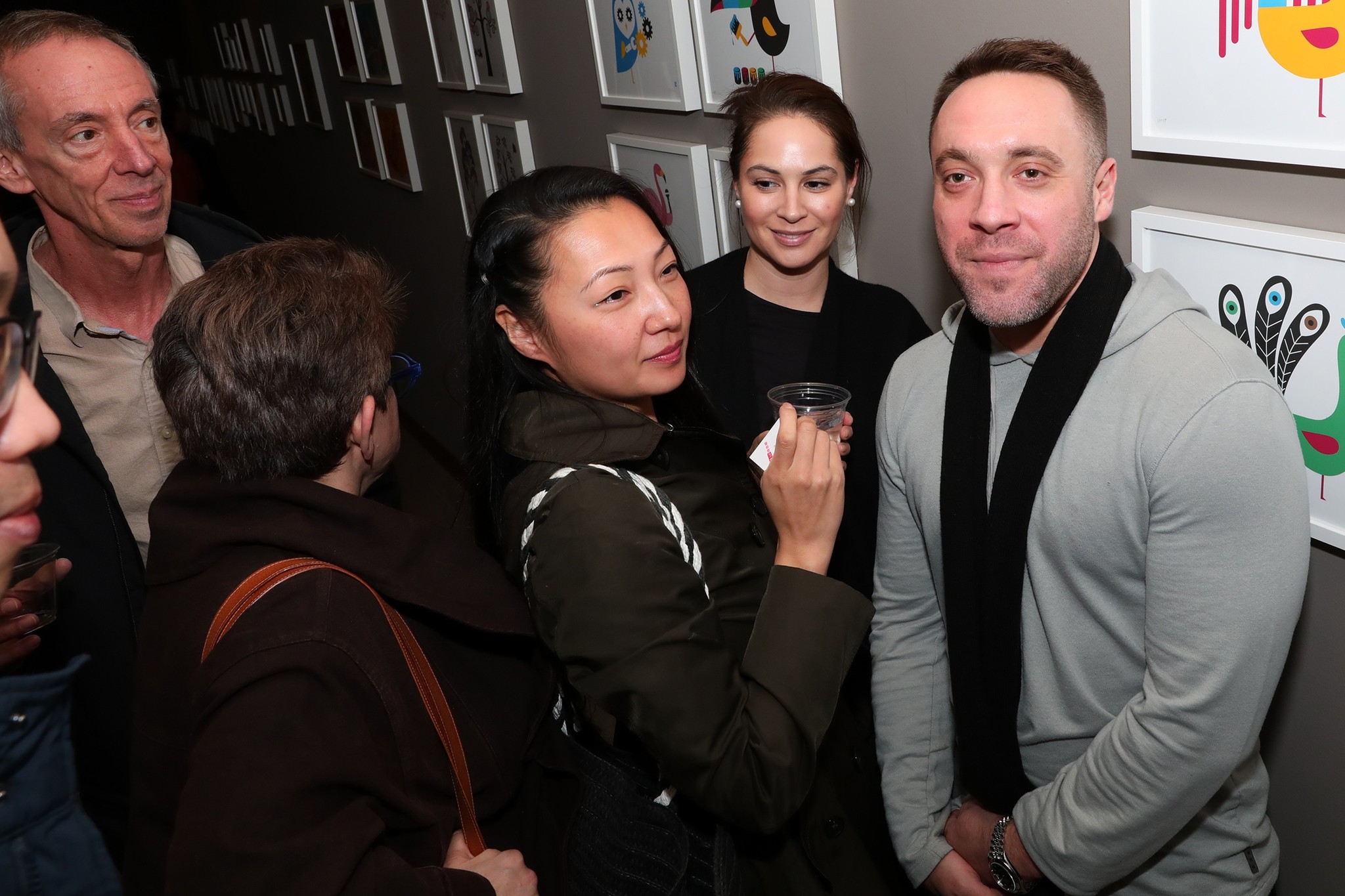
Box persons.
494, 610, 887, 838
159, 95, 231, 213
125, 238, 558, 896
873, 37, 1311, 896
0, 210, 124, 896
470, 167, 871, 896
654, 73, 939, 600
0, 8, 269, 843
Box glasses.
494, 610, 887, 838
2, 309, 44, 415
372, 354, 423, 398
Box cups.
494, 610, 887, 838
3, 542, 61, 636
768, 382, 852, 446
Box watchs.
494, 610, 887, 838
988, 815, 1033, 896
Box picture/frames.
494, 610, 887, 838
480, 115, 536, 192
167, 0, 423, 192
1130, 0, 1345, 169
585, 0, 701, 111
606, 132, 719, 272
708, 146, 859, 280
689, 0, 844, 114
443, 110, 494, 237
1130, 205, 1345, 550
423, 0, 475, 91
459, 0, 524, 95
